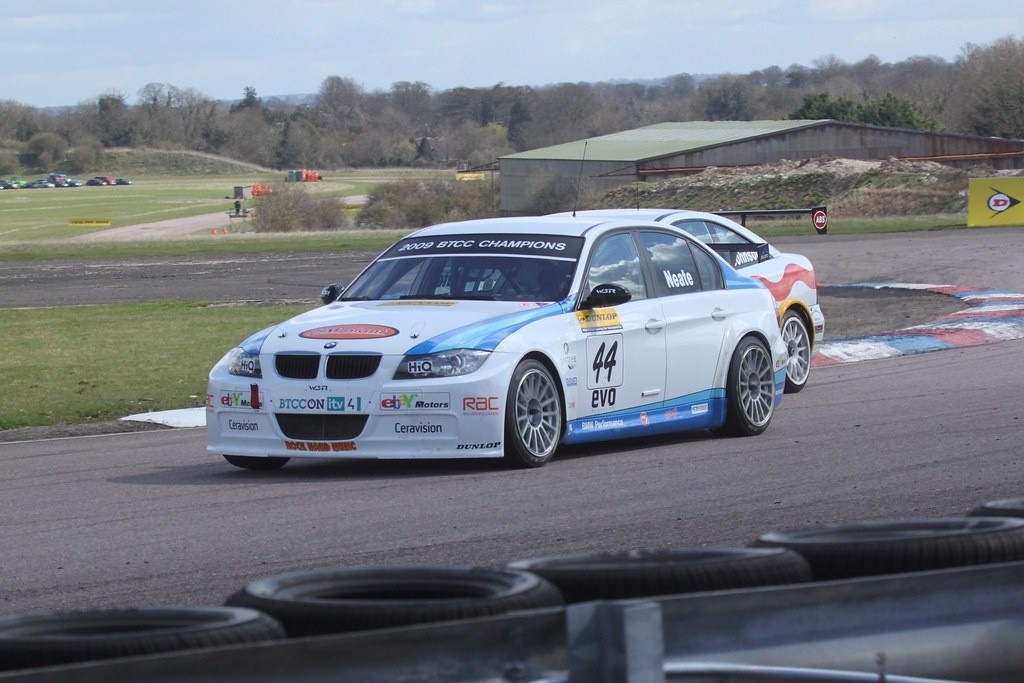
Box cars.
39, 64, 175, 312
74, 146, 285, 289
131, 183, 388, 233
536, 203, 838, 396
196, 215, 787, 468
0, 171, 132, 190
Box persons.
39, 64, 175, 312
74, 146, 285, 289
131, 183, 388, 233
235, 200, 241, 217
503, 256, 577, 304
